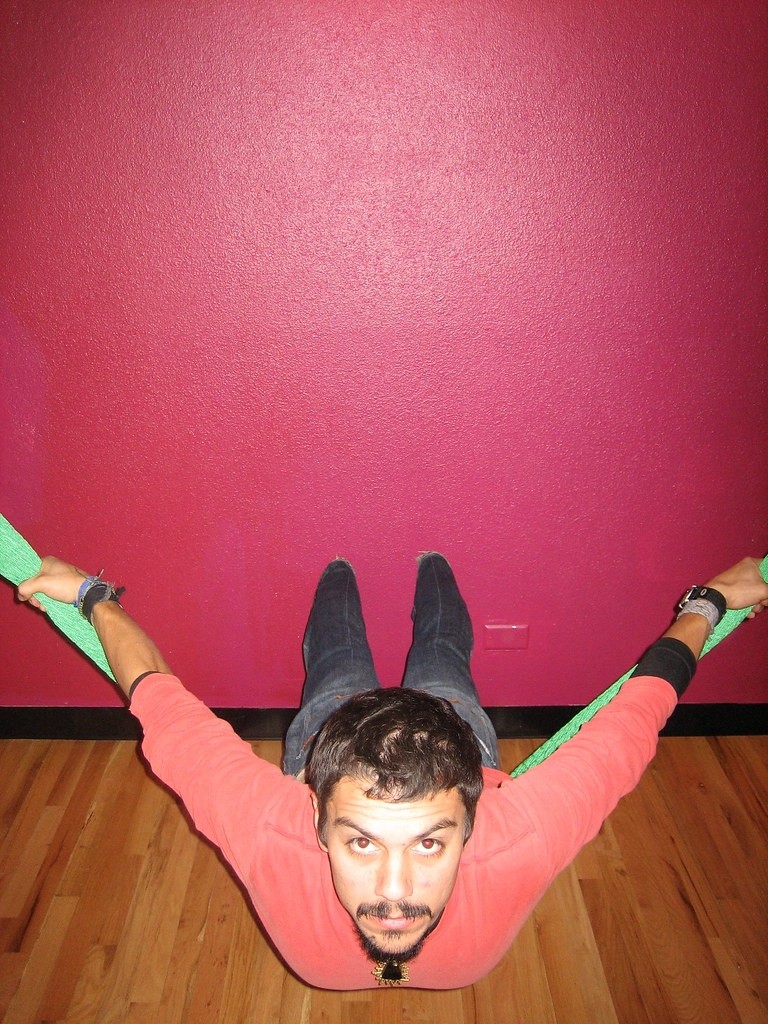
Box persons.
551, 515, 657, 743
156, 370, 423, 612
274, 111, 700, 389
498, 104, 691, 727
15, 528, 768, 992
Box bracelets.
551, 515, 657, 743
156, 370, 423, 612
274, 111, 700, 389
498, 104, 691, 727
675, 600, 719, 630
76, 571, 122, 626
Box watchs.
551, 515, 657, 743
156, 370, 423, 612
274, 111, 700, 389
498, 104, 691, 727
678, 584, 726, 627
